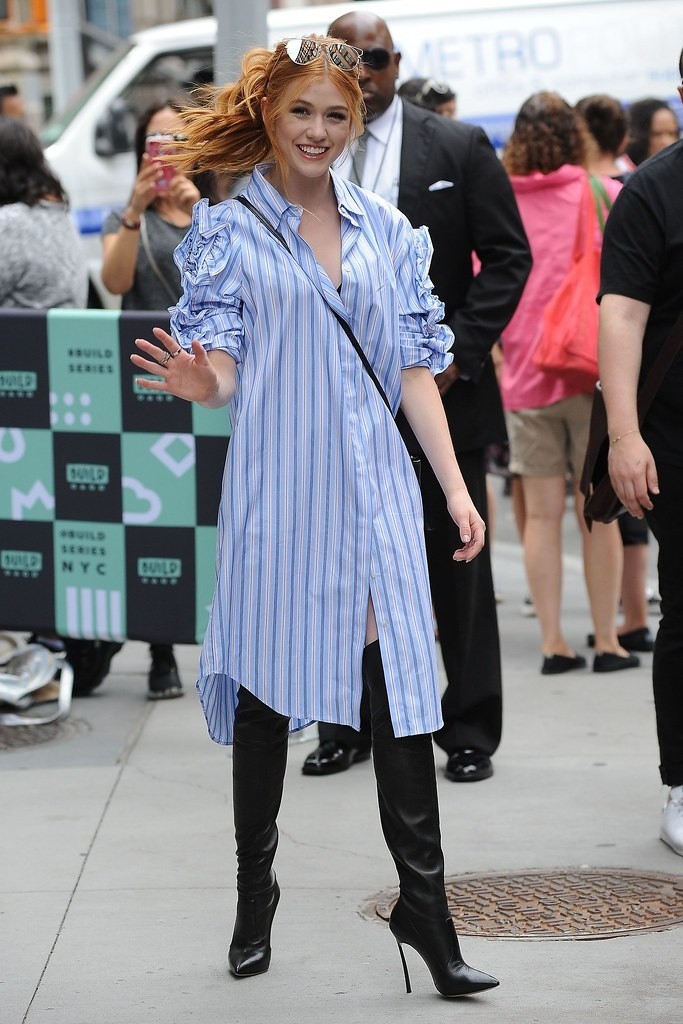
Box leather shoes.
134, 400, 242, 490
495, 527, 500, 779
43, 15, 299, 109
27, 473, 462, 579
446, 749, 493, 782
301, 740, 370, 775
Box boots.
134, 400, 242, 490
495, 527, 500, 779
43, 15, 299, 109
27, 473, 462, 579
362, 639, 501, 997
227, 686, 280, 979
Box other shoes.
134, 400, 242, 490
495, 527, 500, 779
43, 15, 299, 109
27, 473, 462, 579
541, 652, 585, 674
659, 791, 682, 855
619, 593, 660, 608
586, 627, 653, 651
522, 595, 536, 615
593, 651, 639, 672
145, 651, 183, 697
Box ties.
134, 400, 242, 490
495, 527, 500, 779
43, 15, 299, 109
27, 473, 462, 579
349, 125, 371, 186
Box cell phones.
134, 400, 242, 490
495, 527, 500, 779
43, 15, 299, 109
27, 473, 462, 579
146, 135, 177, 190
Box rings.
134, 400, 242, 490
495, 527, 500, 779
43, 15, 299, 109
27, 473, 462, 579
159, 354, 170, 365
170, 347, 183, 357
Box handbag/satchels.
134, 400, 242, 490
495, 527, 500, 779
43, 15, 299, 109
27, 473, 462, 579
2, 632, 75, 727
533, 178, 611, 382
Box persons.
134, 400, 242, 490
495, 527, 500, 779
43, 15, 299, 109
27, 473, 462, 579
129, 35, 502, 999
0, 71, 218, 698
397, 76, 682, 674
301, 11, 534, 779
593, 57, 683, 859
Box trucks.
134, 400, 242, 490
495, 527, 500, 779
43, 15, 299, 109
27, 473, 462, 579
29, 0, 683, 238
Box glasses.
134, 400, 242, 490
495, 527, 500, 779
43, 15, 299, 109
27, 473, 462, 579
262, 37, 365, 96
359, 48, 395, 71
414, 78, 448, 104
